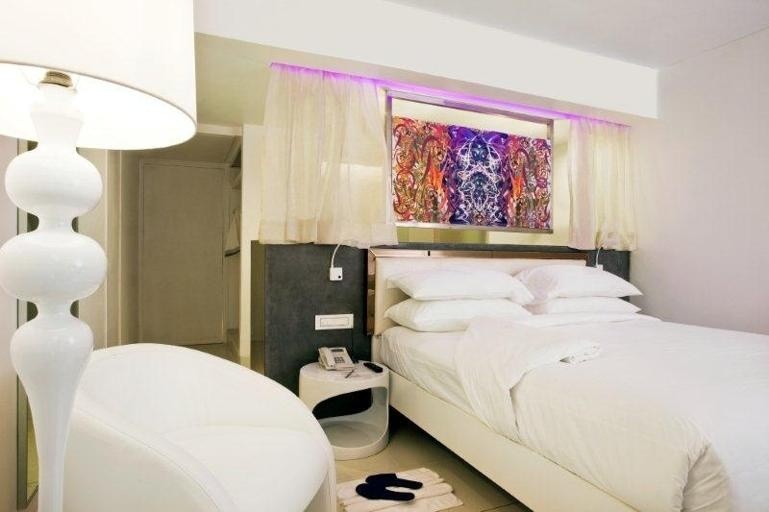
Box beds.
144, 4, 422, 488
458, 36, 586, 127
363, 248, 768, 512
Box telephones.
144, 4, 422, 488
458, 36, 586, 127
318, 347, 354, 371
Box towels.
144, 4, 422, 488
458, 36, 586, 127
556, 343, 605, 366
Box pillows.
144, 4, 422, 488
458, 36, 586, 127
383, 294, 533, 333
515, 261, 646, 301
523, 293, 643, 317
383, 267, 535, 303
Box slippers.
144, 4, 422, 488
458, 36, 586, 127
365, 473, 423, 490
355, 482, 415, 501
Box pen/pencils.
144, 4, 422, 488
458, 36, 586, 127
346, 369, 355, 378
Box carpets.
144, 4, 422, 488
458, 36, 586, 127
332, 466, 464, 511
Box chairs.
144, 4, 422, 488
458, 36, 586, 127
58, 341, 338, 512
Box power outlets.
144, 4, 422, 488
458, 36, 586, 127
328, 265, 344, 283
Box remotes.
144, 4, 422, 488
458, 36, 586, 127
364, 363, 382, 372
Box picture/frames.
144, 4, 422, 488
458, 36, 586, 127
384, 86, 558, 233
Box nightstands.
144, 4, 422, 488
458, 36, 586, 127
296, 357, 392, 460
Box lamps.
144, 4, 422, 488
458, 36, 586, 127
0, 0, 202, 512
